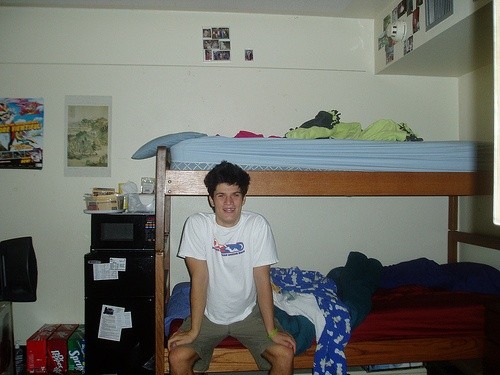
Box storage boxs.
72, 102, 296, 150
47, 324, 79, 375
26, 324, 61, 375
67, 324, 85, 375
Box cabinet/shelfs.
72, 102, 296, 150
84, 251, 155, 375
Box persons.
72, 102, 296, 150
168, 161, 297, 375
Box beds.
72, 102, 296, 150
155, 137, 500, 375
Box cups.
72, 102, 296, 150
141, 177, 155, 194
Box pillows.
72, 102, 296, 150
131, 132, 208, 159
425, 261, 500, 295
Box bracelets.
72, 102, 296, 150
267, 326, 279, 339
